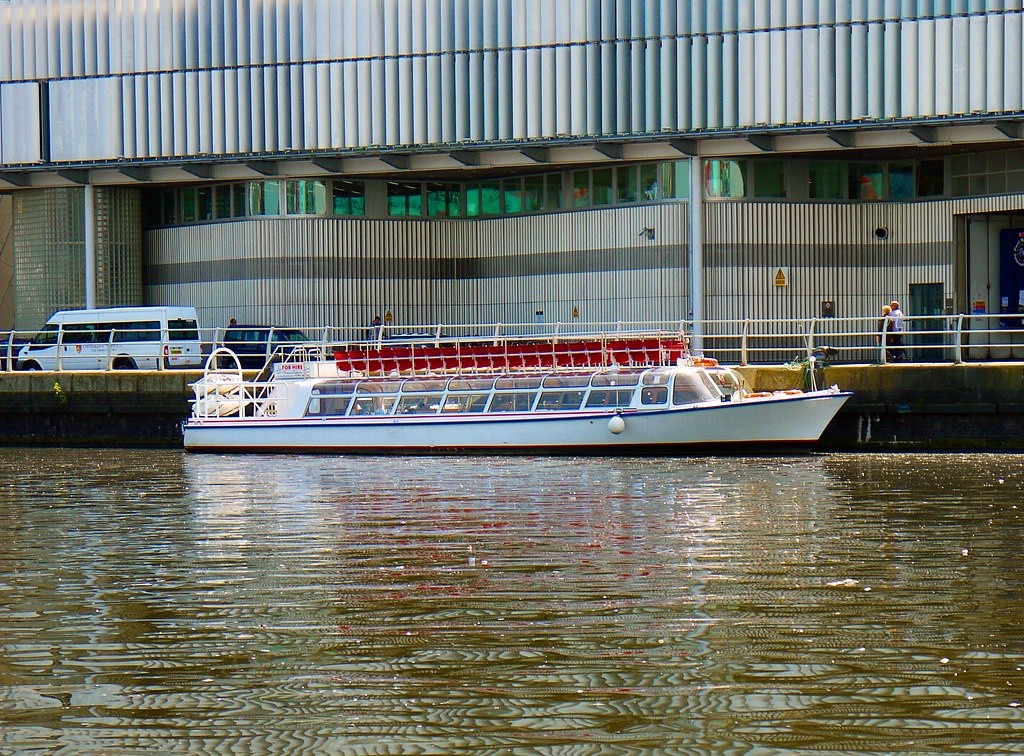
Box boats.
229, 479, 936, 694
179, 366, 853, 453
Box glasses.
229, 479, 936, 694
890, 305, 894, 306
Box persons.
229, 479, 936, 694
877, 301, 904, 362
227, 319, 236, 328
369, 316, 381, 340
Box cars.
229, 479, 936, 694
220, 325, 328, 368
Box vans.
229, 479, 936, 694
16, 304, 203, 369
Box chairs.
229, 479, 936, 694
335, 337, 689, 376
